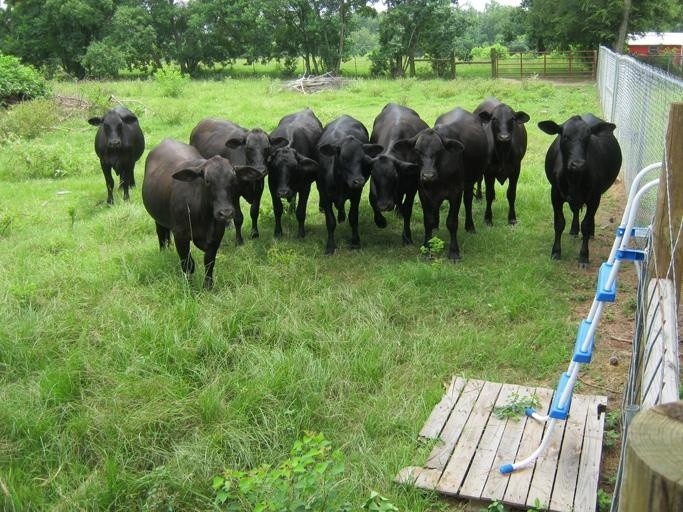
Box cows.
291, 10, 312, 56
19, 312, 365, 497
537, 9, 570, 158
140, 137, 266, 293
87, 105, 146, 206
536, 111, 624, 271
